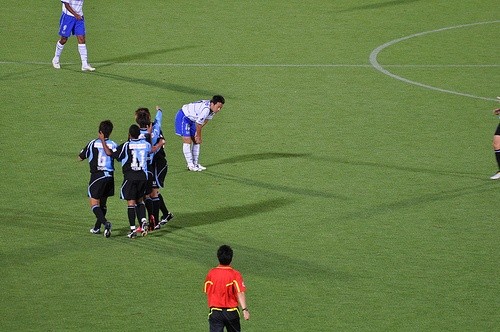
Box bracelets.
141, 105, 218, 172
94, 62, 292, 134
242, 307, 248, 311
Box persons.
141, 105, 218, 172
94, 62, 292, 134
490, 108, 500, 180
135, 108, 174, 225
136, 103, 162, 231
174, 95, 225, 171
99, 124, 165, 238
78, 119, 117, 238
204, 245, 250, 332
51, 0, 96, 72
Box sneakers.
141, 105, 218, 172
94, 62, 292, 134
82, 65, 95, 72
149, 215, 155, 231
103, 222, 112, 238
136, 226, 142, 232
90, 228, 100, 234
140, 218, 148, 236
188, 164, 206, 172
490, 171, 500, 180
126, 228, 137, 239
52, 58, 60, 68
159, 212, 174, 225
154, 224, 160, 229
